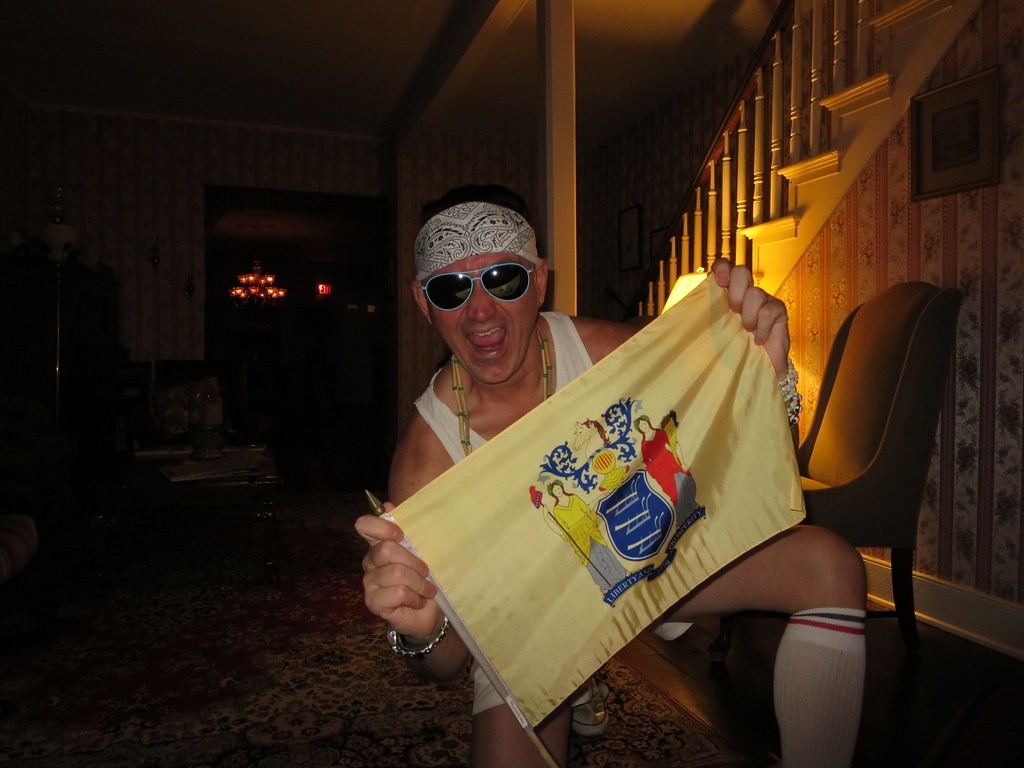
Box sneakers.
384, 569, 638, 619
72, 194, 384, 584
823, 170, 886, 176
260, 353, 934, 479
571, 676, 609, 737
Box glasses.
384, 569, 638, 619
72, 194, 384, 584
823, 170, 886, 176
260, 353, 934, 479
420, 262, 536, 311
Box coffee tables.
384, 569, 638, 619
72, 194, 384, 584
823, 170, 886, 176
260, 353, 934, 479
86, 457, 284, 595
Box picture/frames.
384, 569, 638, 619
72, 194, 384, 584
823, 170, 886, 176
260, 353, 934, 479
906, 64, 1002, 204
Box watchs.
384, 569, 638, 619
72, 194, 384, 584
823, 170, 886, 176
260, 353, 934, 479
385, 614, 450, 658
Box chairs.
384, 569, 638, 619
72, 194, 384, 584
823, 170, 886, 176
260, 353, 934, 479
707, 279, 963, 666
120, 355, 267, 454
0, 463, 58, 631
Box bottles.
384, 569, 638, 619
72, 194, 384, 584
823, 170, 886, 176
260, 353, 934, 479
52, 186, 64, 222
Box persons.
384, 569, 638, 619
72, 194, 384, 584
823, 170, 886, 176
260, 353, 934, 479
355, 185, 869, 768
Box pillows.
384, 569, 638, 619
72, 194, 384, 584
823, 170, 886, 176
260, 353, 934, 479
154, 373, 226, 438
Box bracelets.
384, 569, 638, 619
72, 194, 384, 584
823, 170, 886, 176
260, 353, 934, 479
777, 356, 803, 427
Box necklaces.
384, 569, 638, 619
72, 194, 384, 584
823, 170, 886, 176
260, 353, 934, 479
451, 323, 556, 460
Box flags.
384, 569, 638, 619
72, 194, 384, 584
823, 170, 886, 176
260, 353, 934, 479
390, 271, 807, 730
